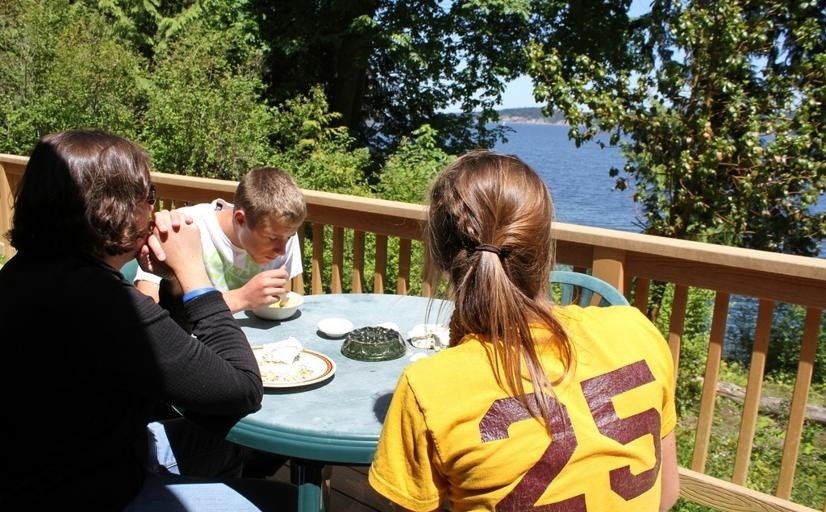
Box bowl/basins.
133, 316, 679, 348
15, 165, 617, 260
317, 317, 353, 338
250, 291, 304, 322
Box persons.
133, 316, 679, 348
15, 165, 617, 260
134, 168, 312, 483
0, 129, 267, 511
364, 153, 680, 512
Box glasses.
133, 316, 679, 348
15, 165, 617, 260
145, 182, 158, 207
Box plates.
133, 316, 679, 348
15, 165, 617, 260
248, 344, 337, 390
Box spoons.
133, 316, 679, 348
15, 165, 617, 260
277, 293, 292, 307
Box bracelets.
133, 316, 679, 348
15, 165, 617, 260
181, 286, 219, 304
